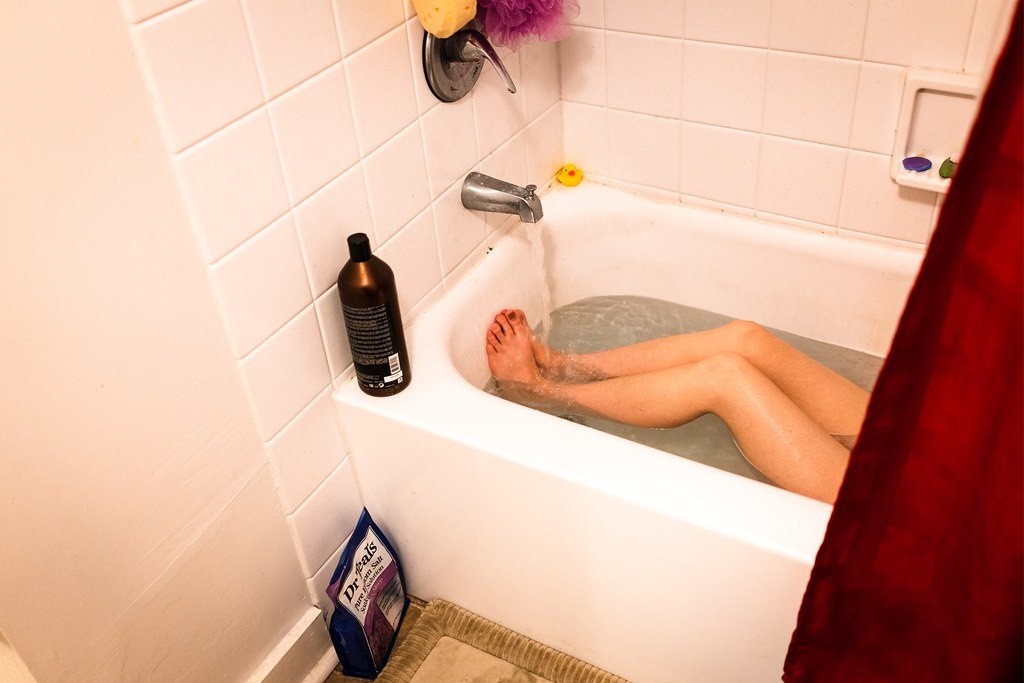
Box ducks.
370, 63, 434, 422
556, 163, 584, 187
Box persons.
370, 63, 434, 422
485, 308, 875, 506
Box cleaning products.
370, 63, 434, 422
325, 508, 411, 680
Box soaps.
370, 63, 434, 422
901, 156, 959, 178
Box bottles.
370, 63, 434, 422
337, 233, 412, 398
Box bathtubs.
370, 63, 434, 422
337, 175, 1023, 683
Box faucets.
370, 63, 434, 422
461, 171, 544, 224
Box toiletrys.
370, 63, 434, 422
336, 233, 412, 398
